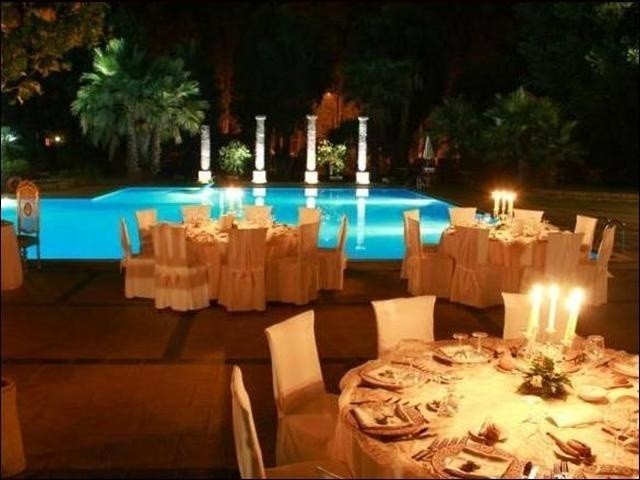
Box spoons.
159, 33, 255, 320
379, 426, 428, 441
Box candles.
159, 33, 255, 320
492, 188, 519, 222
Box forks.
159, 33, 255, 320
413, 436, 470, 461
552, 461, 569, 479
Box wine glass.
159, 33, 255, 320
449, 334, 468, 369
470, 332, 488, 367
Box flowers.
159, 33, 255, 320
514, 344, 575, 401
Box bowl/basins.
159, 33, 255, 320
575, 385, 610, 401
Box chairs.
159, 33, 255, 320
400, 204, 616, 308
231, 366, 352, 478
266, 309, 340, 466
373, 296, 435, 355
501, 292, 574, 338
119, 204, 348, 312
14, 177, 42, 275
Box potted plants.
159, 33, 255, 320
218, 135, 253, 189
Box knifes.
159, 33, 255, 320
521, 462, 540, 479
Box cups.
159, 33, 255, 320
588, 335, 604, 361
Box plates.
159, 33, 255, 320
430, 342, 494, 365
552, 445, 596, 461
445, 446, 514, 480
545, 402, 606, 427
359, 366, 419, 387
340, 404, 425, 436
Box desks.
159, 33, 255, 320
339, 334, 639, 479
